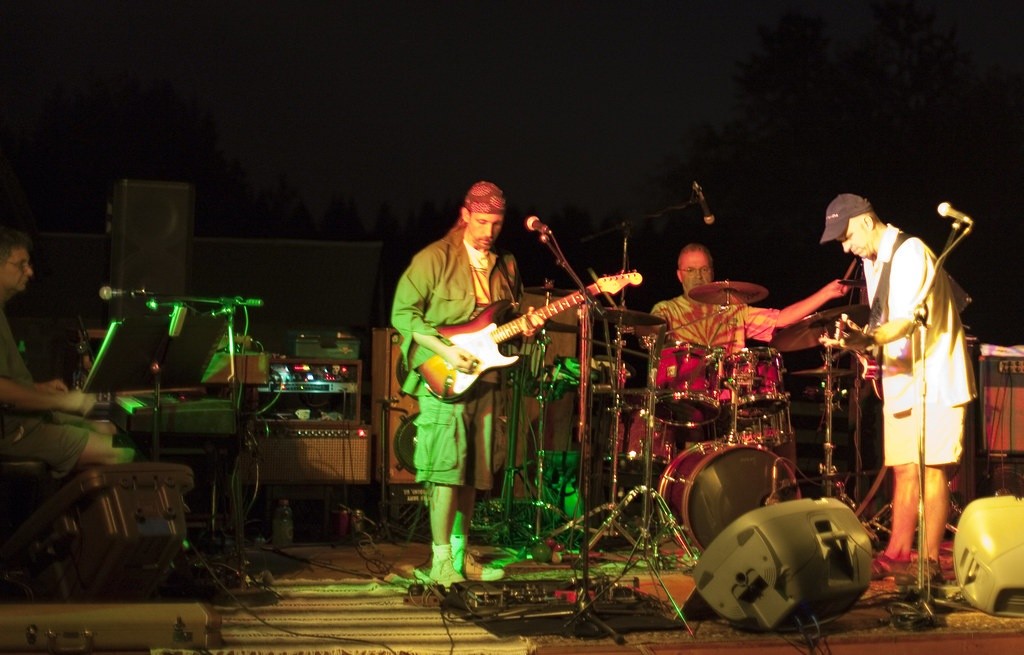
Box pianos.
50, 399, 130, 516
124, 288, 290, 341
106, 386, 257, 594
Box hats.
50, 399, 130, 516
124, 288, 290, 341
818, 192, 872, 244
464, 181, 507, 214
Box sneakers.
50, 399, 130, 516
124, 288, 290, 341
905, 556, 944, 587
452, 550, 505, 581
866, 553, 903, 581
430, 555, 466, 588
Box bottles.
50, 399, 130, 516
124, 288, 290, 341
273, 499, 294, 547
351, 509, 365, 532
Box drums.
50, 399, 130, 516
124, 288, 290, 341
602, 405, 674, 475
649, 340, 721, 427
657, 440, 801, 554
727, 347, 790, 420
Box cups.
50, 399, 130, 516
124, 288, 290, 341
331, 510, 348, 547
295, 409, 310, 420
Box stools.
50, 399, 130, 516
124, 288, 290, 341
0, 458, 44, 515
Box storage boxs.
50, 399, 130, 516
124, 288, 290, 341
287, 327, 362, 358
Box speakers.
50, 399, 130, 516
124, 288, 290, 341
693, 498, 873, 632
370, 325, 430, 507
0, 463, 194, 606
980, 354, 1024, 457
247, 418, 372, 485
489, 295, 580, 501
953, 495, 1024, 618
110, 178, 196, 316
977, 454, 1024, 499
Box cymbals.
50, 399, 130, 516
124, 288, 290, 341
788, 368, 854, 378
768, 304, 870, 352
593, 306, 667, 328
688, 281, 770, 305
838, 280, 868, 288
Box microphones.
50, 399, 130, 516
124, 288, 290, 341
98, 285, 147, 301
692, 181, 714, 225
527, 216, 552, 236
766, 462, 781, 506
938, 202, 974, 223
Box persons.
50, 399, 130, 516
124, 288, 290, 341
618, 243, 849, 450
0, 227, 137, 481
819, 194, 978, 587
390, 182, 545, 587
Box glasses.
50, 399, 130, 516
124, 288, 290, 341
677, 266, 712, 276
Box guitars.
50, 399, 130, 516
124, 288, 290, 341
834, 313, 884, 400
415, 269, 645, 404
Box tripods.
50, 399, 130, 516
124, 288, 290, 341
482, 235, 700, 646
855, 221, 979, 629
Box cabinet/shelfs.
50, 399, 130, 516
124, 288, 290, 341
222, 360, 362, 537
